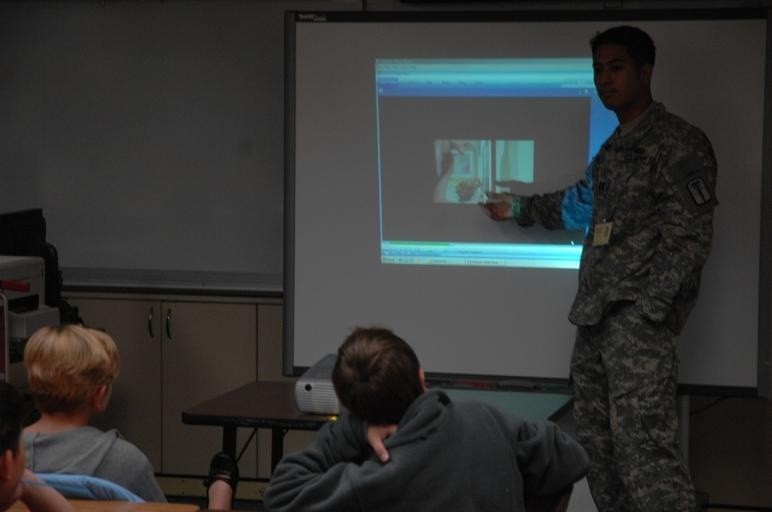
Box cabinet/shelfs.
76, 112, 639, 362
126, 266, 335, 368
60, 288, 283, 500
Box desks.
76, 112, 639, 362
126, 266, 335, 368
181, 381, 572, 498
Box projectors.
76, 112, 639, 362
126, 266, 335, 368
295, 353, 345, 416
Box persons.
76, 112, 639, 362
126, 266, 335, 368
261, 322, 591, 511
0, 379, 80, 512
474, 22, 719, 511
15, 323, 173, 505
